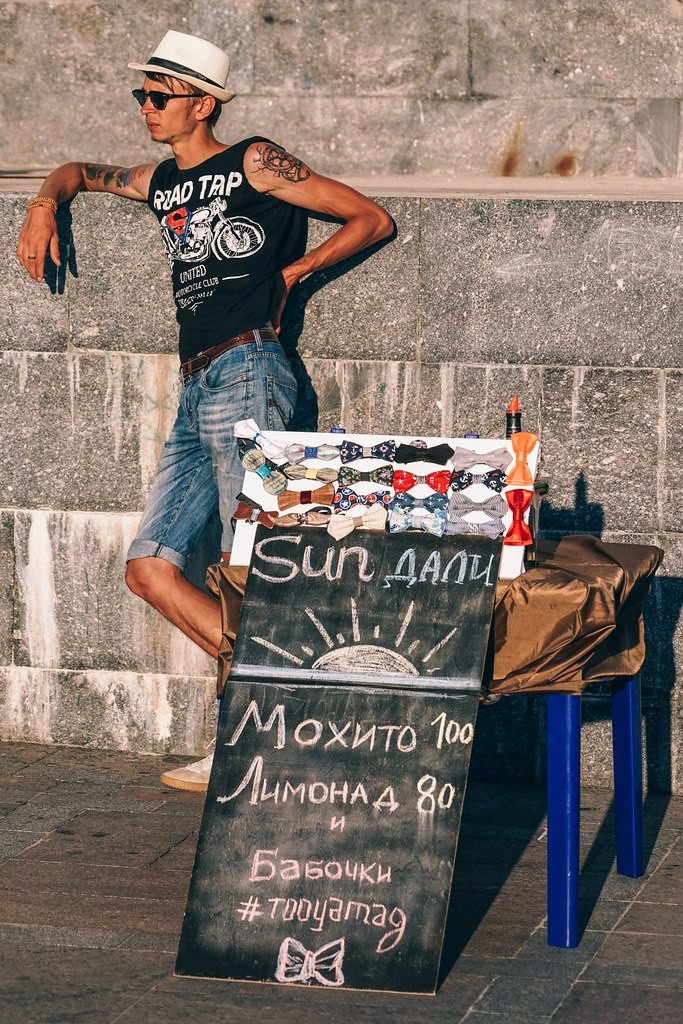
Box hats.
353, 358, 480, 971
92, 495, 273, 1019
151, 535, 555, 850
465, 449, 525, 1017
127, 28, 238, 105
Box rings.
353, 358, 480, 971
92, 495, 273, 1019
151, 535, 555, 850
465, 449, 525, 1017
27, 257, 36, 259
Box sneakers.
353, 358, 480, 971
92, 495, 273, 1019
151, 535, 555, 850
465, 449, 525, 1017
160, 750, 215, 792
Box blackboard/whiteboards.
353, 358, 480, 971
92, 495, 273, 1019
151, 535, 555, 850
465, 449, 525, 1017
169, 522, 508, 997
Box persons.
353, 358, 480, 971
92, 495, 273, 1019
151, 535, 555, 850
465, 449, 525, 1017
16, 31, 395, 792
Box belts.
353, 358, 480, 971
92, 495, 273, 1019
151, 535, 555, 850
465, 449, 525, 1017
178, 330, 280, 382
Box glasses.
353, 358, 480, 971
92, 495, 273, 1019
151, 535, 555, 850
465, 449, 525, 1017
132, 89, 201, 111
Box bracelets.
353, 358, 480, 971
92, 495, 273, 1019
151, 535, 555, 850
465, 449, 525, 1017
28, 197, 58, 214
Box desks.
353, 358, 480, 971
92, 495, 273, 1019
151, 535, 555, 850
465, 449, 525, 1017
205, 535, 664, 947
